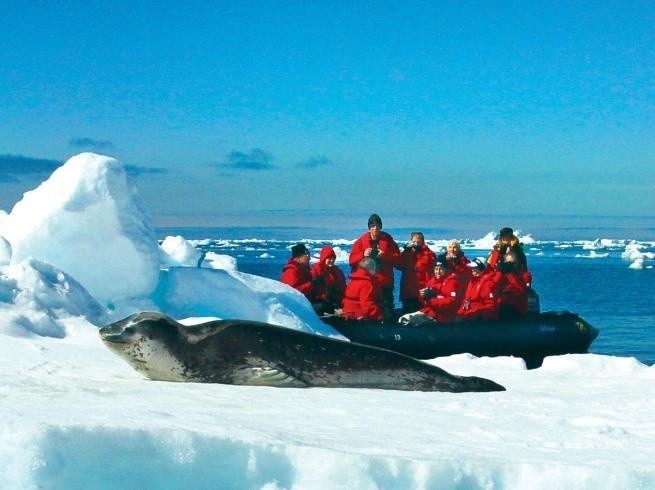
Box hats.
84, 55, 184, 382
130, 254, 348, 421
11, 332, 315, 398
291, 243, 309, 259
465, 256, 488, 272
368, 213, 383, 229
433, 253, 447, 268
320, 245, 336, 263
499, 227, 513, 236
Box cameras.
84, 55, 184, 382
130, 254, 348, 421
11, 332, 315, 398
369, 238, 379, 258
403, 242, 419, 253
424, 289, 436, 300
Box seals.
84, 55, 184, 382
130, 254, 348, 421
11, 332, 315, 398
98, 311, 506, 393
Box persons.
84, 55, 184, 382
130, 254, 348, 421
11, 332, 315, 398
280, 214, 532, 326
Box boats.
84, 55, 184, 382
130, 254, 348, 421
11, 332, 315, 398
320, 307, 600, 369
